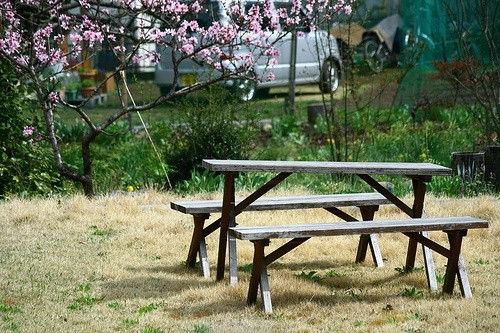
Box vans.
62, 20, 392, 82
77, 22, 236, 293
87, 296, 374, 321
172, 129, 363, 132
156, 0, 343, 103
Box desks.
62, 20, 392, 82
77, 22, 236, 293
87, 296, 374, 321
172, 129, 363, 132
202, 156, 453, 295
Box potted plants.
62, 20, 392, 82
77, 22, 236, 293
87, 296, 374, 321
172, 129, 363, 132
65, 66, 116, 102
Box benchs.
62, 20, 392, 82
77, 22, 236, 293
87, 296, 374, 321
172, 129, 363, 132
228, 214, 490, 312
170, 191, 394, 278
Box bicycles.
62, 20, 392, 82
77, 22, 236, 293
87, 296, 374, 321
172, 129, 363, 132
363, 16, 435, 74
